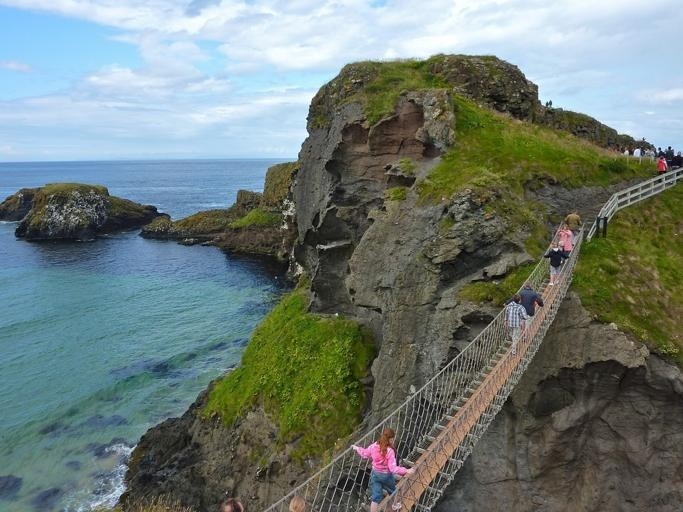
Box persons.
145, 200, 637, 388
557, 224, 573, 258
564, 209, 582, 237
621, 145, 673, 183
504, 295, 530, 354
544, 242, 570, 286
350, 428, 415, 512
503, 282, 544, 339
289, 495, 306, 512
557, 242, 565, 271
219, 497, 244, 512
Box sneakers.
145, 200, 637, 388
392, 502, 402, 511
